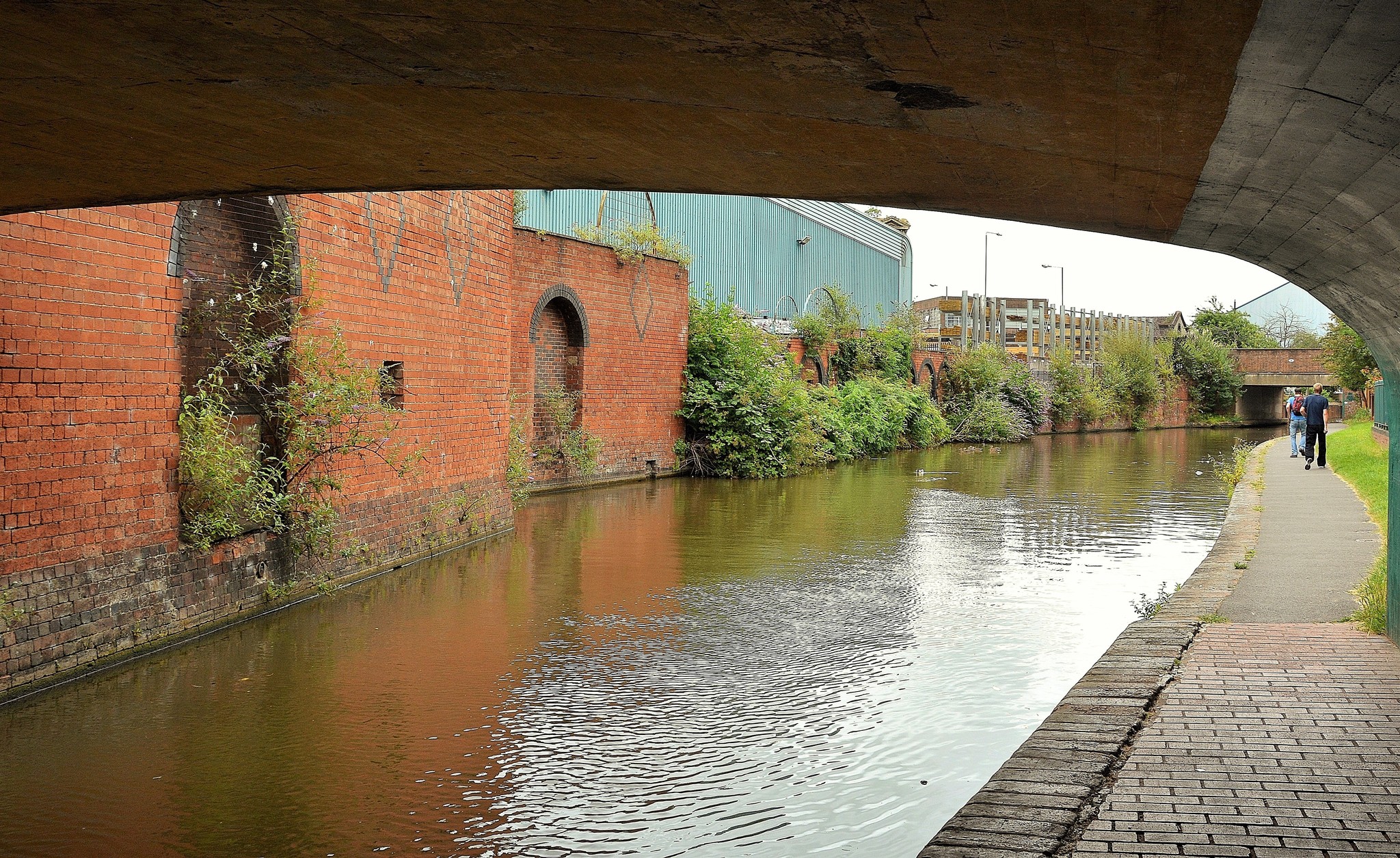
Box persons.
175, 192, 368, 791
1286, 388, 1306, 458
1300, 382, 1329, 471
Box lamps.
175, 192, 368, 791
796, 236, 812, 244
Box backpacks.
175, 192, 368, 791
1291, 396, 1305, 416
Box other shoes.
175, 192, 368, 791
1290, 454, 1297, 457
1299, 449, 1305, 456
1318, 465, 1326, 468
1305, 458, 1312, 470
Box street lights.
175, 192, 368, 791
1042, 264, 1064, 306
929, 284, 948, 301
985, 232, 1002, 297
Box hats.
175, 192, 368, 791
1294, 388, 1302, 392
